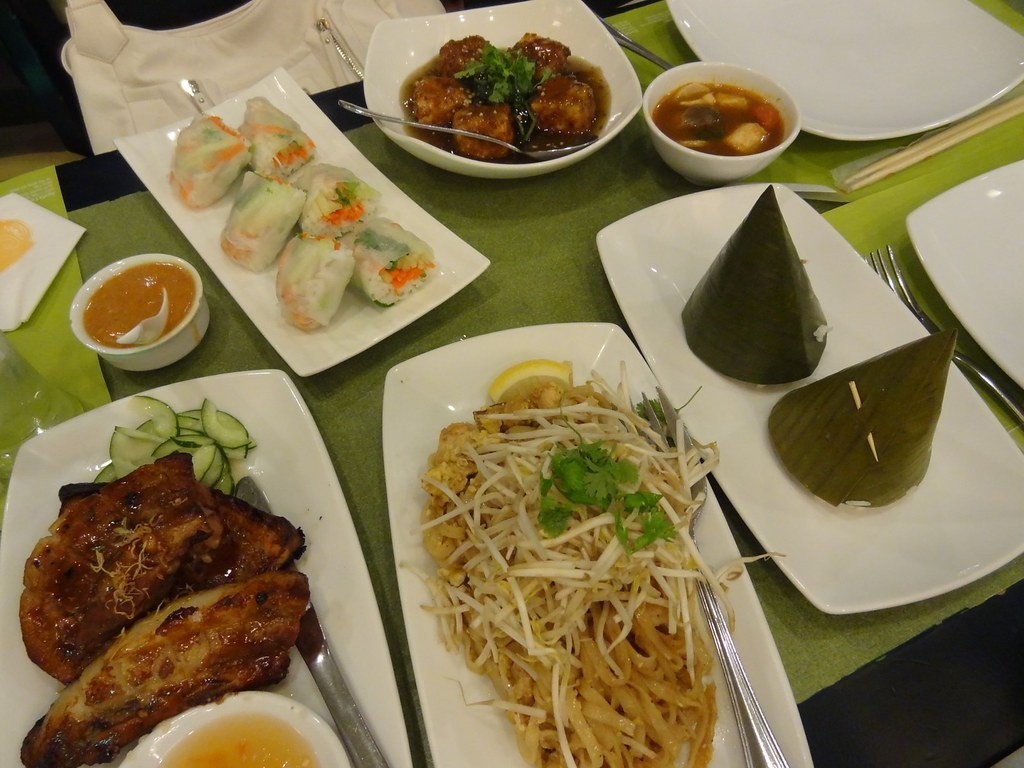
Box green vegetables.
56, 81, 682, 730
453, 40, 554, 142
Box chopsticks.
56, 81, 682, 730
843, 95, 1024, 192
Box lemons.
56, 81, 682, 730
488, 359, 574, 403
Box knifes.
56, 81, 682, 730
233, 475, 389, 768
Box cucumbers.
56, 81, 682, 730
91, 394, 255, 497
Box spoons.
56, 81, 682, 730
116, 288, 169, 345
338, 99, 599, 160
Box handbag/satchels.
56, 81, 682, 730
55, 0, 447, 156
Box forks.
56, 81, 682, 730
863, 243, 1024, 427
628, 385, 788, 768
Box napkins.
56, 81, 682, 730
0, 164, 110, 531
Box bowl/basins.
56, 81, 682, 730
643, 60, 802, 188
363, 0, 644, 180
70, 254, 210, 370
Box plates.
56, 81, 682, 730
904, 158, 1024, 389
111, 66, 491, 377
664, 0, 1024, 142
117, 691, 350, 768
382, 322, 814, 768
0, 369, 413, 768
596, 183, 1024, 615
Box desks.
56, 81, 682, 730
58, 0, 1024, 768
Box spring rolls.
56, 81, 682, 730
169, 96, 435, 331
